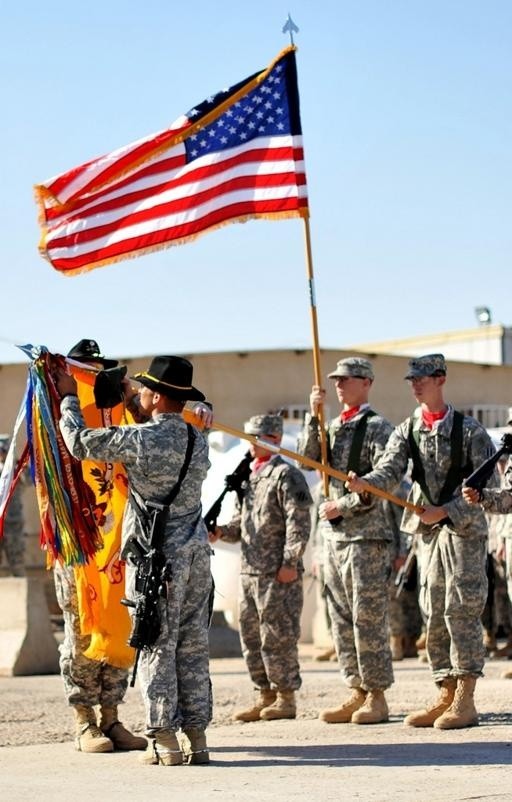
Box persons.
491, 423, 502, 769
295, 357, 408, 724
345, 353, 501, 729
53, 356, 214, 766
53, 339, 149, 754
314, 419, 512, 681
208, 413, 314, 722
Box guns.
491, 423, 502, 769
462, 419, 512, 499
205, 407, 287, 533
121, 499, 170, 652
393, 541, 418, 600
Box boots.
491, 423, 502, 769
73, 703, 113, 752
179, 728, 210, 764
139, 731, 185, 766
433, 676, 478, 731
415, 631, 512, 660
403, 676, 456, 727
99, 704, 148, 751
351, 688, 389, 726
315, 634, 405, 662
321, 686, 367, 724
259, 689, 297, 721
235, 688, 275, 720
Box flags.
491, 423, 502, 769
32, 45, 309, 277
0, 351, 205, 669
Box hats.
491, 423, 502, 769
242, 414, 284, 438
130, 354, 206, 403
403, 353, 448, 381
327, 356, 376, 381
68, 338, 118, 370
